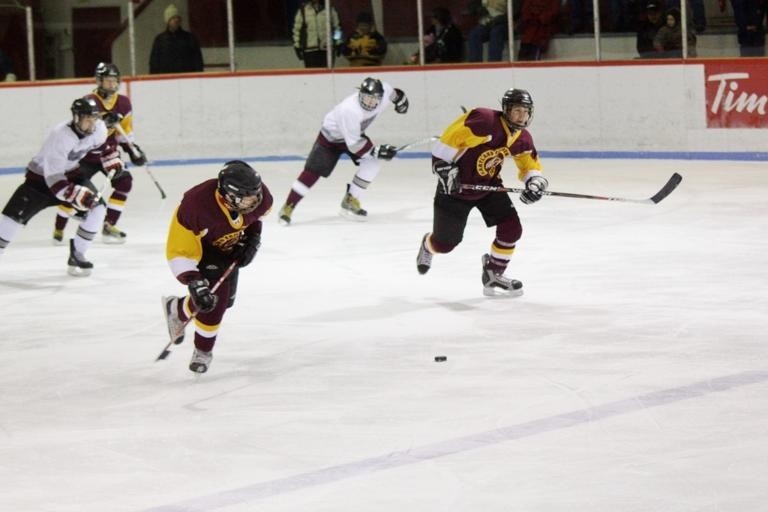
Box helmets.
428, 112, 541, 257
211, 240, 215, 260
501, 88, 534, 130
218, 161, 263, 215
358, 78, 383, 111
95, 62, 120, 99
71, 99, 100, 137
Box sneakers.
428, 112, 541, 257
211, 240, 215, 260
102, 222, 126, 237
54, 228, 63, 240
190, 347, 213, 373
165, 295, 184, 344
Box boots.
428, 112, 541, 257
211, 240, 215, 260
279, 201, 293, 222
341, 183, 367, 216
68, 239, 93, 268
417, 233, 432, 274
481, 254, 522, 290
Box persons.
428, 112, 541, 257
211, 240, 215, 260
164, 160, 273, 373
731, 2, 767, 57
0, 98, 124, 268
290, 1, 387, 68
149, 4, 204, 74
635, 2, 706, 59
411, 1, 563, 68
280, 78, 409, 223
417, 88, 550, 290
54, 62, 145, 241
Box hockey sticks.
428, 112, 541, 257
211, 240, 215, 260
393, 105, 469, 155
58, 166, 116, 220
158, 263, 239, 360
461, 173, 682, 204
112, 120, 169, 203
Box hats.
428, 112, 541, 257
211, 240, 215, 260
163, 3, 181, 26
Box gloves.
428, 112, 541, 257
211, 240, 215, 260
63, 181, 102, 212
188, 280, 217, 314
229, 231, 261, 267
392, 88, 409, 113
370, 144, 397, 159
432, 160, 463, 196
100, 151, 125, 180
128, 144, 148, 166
519, 175, 548, 205
